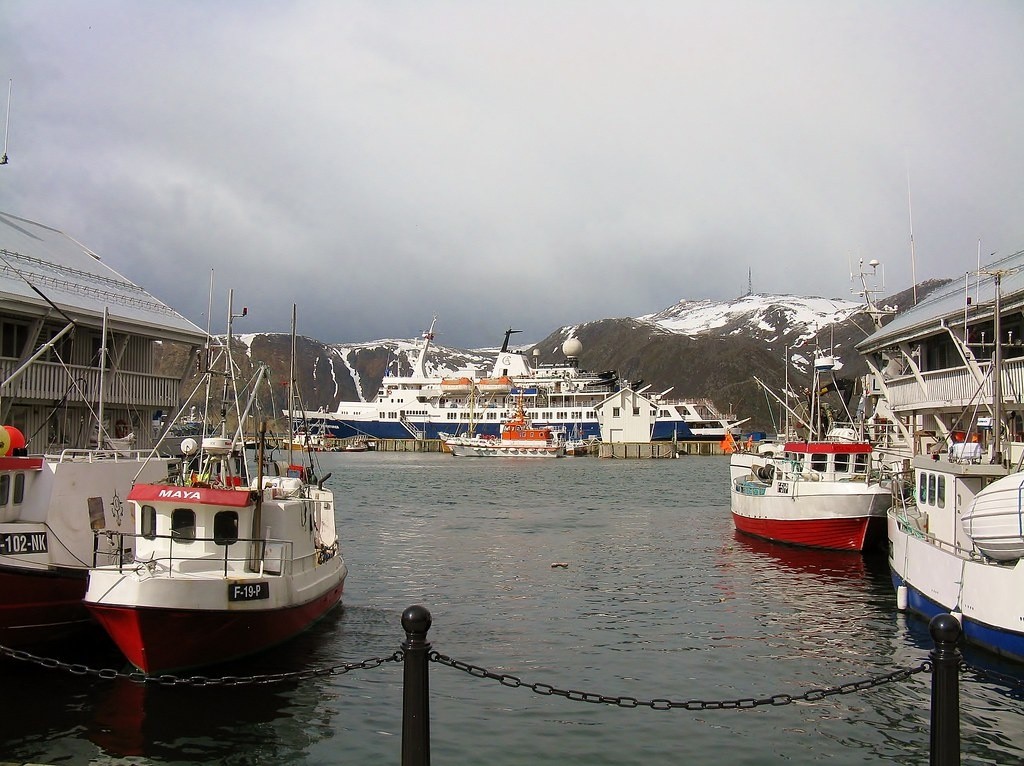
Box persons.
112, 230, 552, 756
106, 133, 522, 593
800, 386, 830, 441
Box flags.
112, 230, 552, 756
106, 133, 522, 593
720, 428, 753, 454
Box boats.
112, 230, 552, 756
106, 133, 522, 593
0, 306, 181, 650
436, 389, 567, 458
281, 314, 753, 442
79, 290, 349, 677
884, 267, 1024, 663
729, 357, 906, 552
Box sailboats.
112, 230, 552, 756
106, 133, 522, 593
281, 406, 368, 452
